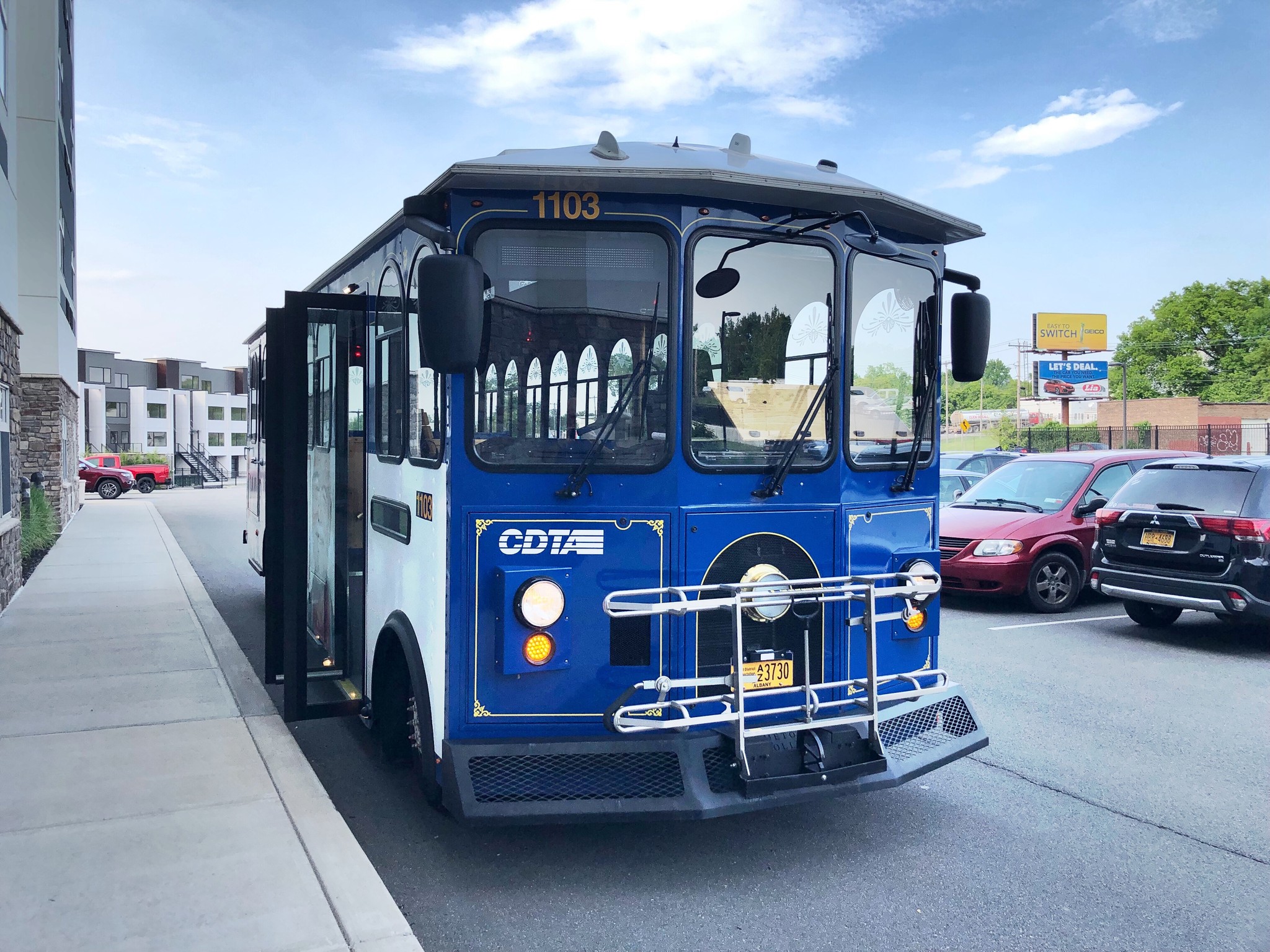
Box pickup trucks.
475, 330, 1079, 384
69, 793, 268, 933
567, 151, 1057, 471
84, 455, 172, 494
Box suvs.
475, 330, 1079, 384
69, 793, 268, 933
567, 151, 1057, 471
1087, 454, 1270, 641
77, 457, 137, 499
939, 449, 1212, 614
938, 446, 1040, 509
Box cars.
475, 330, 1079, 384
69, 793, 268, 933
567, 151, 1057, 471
1044, 379, 1075, 395
1055, 442, 1109, 453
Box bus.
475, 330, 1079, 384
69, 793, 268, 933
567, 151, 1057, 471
242, 130, 992, 826
